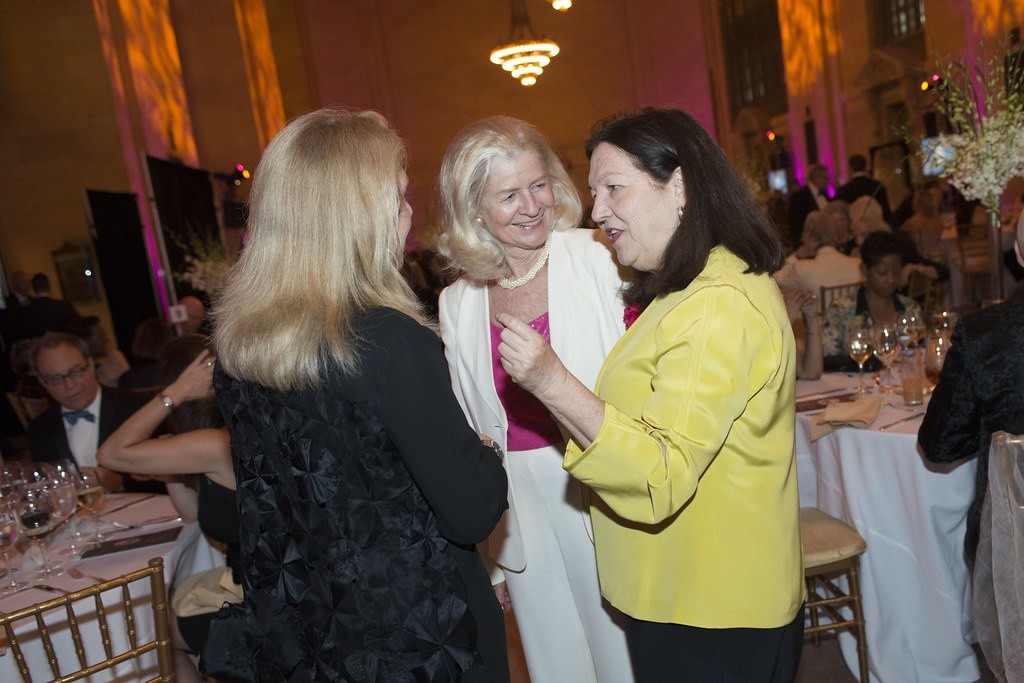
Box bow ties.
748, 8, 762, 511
63, 410, 95, 426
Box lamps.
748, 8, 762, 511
488, 0, 560, 88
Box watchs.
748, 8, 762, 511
157, 393, 177, 412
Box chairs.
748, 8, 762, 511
0, 557, 176, 683
798, 507, 869, 683
820, 281, 865, 357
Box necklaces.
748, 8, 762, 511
494, 227, 553, 291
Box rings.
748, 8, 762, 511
207, 362, 212, 367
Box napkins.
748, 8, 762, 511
811, 395, 883, 441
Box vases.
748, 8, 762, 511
985, 209, 1008, 309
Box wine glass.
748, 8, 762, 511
0, 461, 112, 594
848, 312, 952, 398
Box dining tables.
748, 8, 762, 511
0, 493, 227, 683
794, 372, 982, 683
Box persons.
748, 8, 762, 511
118, 296, 206, 403
0, 270, 110, 399
917, 209, 1024, 598
494, 103, 809, 683
438, 114, 635, 683
207, 104, 510, 683
27, 331, 170, 495
96, 348, 239, 683
771, 153, 980, 381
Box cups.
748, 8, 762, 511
925, 340, 951, 385
899, 349, 924, 406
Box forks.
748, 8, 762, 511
884, 403, 916, 411
113, 515, 181, 528
68, 567, 108, 583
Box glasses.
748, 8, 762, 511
37, 363, 89, 386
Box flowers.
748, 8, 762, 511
907, 26, 1024, 212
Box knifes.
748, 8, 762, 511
33, 584, 72, 595
878, 412, 926, 431
102, 518, 181, 535
97, 493, 156, 518
795, 388, 846, 399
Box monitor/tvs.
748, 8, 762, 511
921, 136, 956, 176
767, 169, 788, 194
